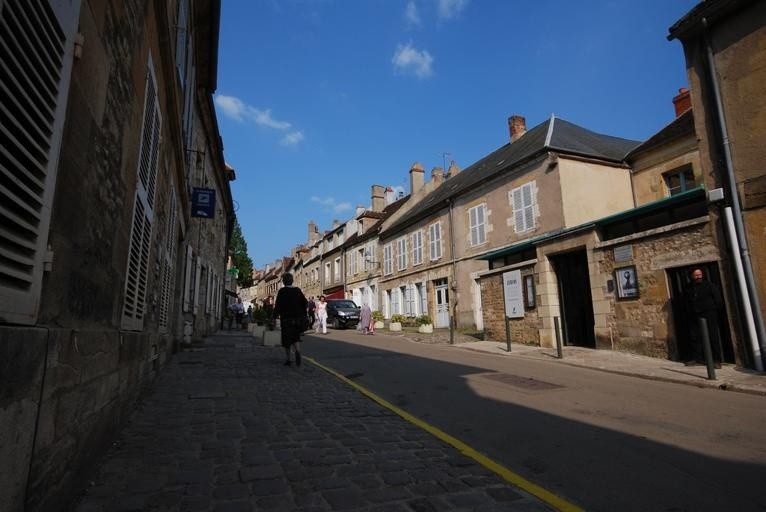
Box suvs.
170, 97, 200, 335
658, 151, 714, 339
325, 299, 361, 329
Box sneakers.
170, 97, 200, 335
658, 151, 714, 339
295, 351, 301, 366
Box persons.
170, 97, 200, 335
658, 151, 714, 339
683, 266, 724, 369
226, 295, 328, 335
272, 272, 308, 367
357, 303, 377, 336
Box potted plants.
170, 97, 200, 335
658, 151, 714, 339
371, 310, 385, 330
415, 314, 434, 334
389, 313, 406, 332
263, 317, 282, 347
253, 310, 267, 338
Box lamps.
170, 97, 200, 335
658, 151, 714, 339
365, 252, 380, 264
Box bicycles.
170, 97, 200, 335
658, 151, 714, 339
229, 309, 239, 331
